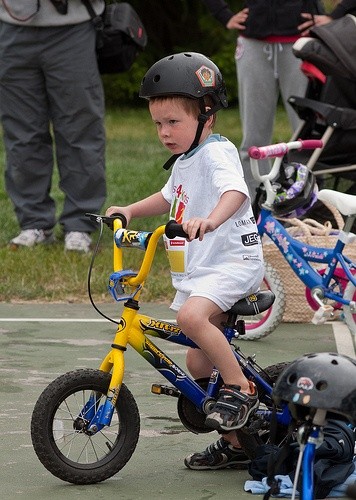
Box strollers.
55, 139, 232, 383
249, 13, 355, 234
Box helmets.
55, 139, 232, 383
138, 51, 229, 110
252, 162, 319, 223
273, 352, 356, 430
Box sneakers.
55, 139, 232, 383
11, 228, 49, 248
64, 231, 92, 252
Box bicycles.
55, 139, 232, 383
28, 211, 311, 485
233, 137, 356, 341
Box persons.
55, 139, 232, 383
105, 53, 265, 471
0, 0, 106, 253
205, 0, 356, 207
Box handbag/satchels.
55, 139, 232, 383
95, 1, 148, 75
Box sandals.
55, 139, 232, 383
204, 381, 260, 431
184, 437, 251, 470
